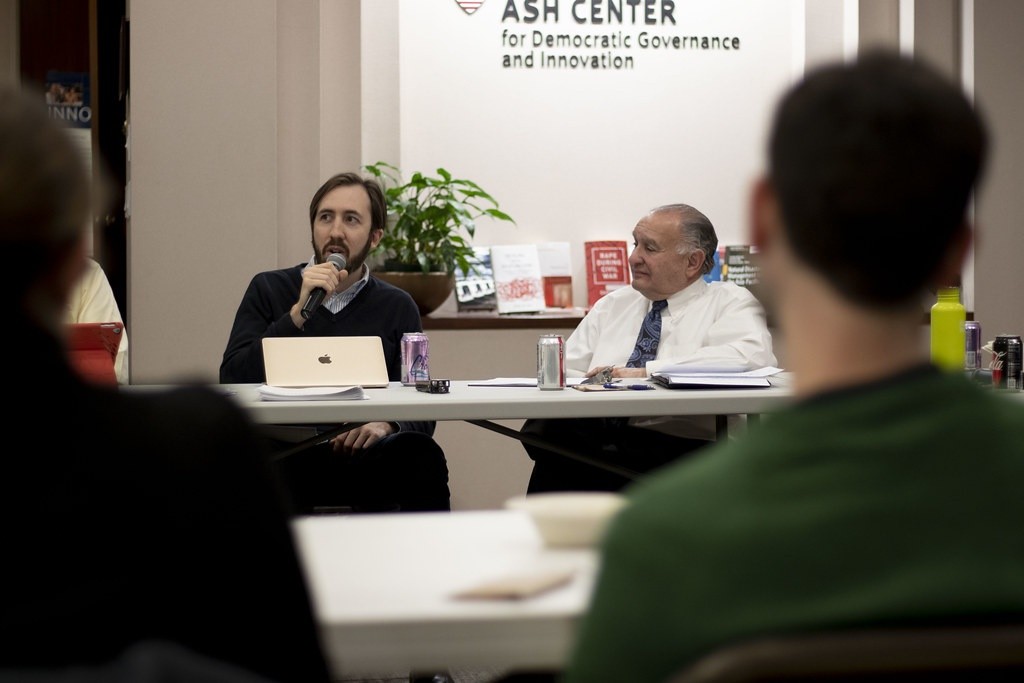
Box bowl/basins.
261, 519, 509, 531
505, 493, 628, 547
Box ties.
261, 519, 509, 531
626, 300, 668, 368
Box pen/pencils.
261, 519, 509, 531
604, 383, 656, 390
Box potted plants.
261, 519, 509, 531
357, 160, 517, 316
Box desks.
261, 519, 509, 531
291, 502, 601, 674
216, 374, 800, 444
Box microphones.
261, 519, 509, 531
300, 253, 346, 320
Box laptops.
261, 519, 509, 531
263, 335, 389, 388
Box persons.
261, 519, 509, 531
528, 204, 778, 494
569, 46, 1023, 682
0, 78, 339, 683
220, 173, 453, 683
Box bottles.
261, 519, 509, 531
930, 278, 967, 373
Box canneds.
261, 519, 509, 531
964, 320, 983, 371
400, 331, 430, 387
537, 334, 566, 391
990, 334, 1023, 393
